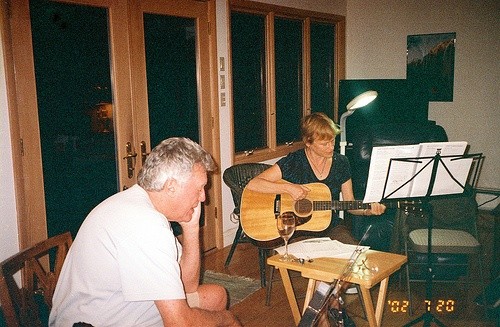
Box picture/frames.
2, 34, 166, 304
406, 32, 456, 102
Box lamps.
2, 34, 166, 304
340, 89, 379, 221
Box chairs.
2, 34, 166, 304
222, 162, 272, 290
401, 198, 492, 325
467, 183, 499, 236
0, 232, 71, 327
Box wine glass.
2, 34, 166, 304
276, 215, 295, 261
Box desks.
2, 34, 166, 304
268, 240, 408, 325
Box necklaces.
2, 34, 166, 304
305, 147, 327, 177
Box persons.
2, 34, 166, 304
247, 113, 387, 327
49, 138, 244, 327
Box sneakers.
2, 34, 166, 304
329, 308, 356, 327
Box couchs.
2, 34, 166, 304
352, 125, 474, 251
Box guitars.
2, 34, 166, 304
239, 182, 424, 249
